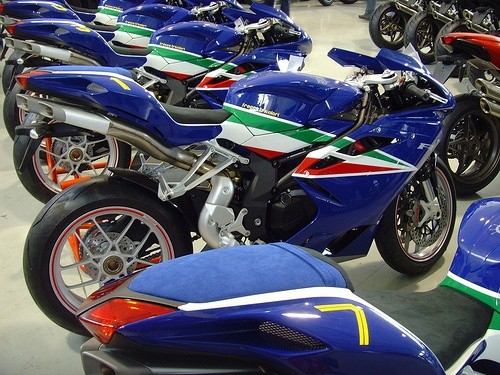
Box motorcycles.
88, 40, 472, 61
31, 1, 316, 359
1, 1, 499, 375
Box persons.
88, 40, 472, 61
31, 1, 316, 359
263, 0, 290, 18
358, 0, 377, 20
